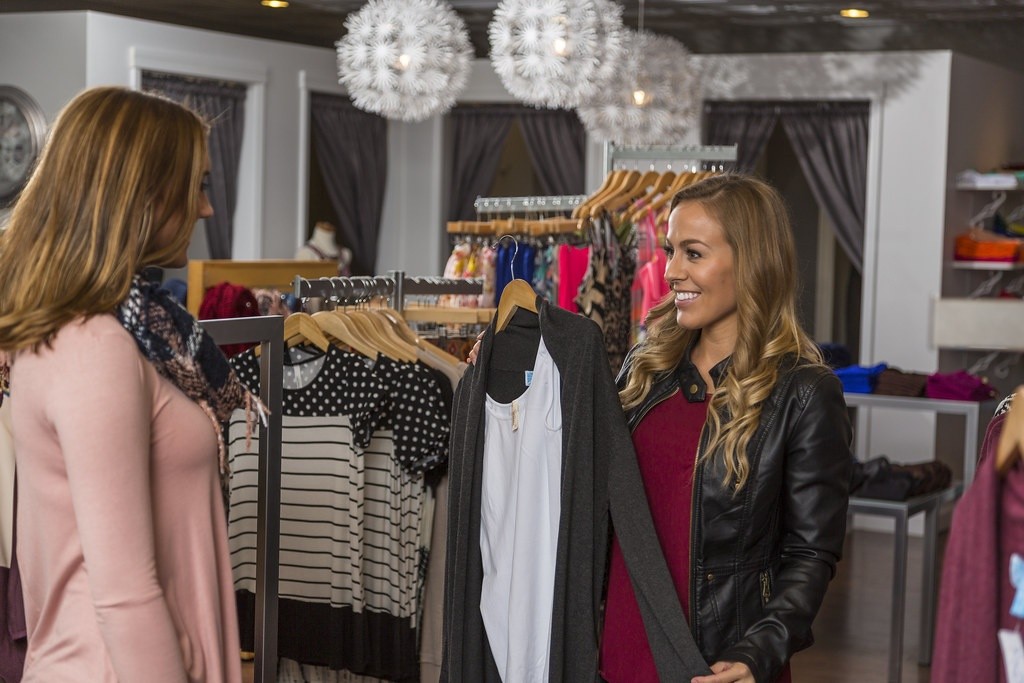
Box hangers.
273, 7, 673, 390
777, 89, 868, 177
254, 140, 727, 366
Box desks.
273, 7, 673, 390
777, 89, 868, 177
844, 392, 1003, 492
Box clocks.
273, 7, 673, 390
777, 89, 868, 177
0, 83, 48, 208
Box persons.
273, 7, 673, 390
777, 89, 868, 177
0, 86, 266, 683
466, 175, 851, 683
296, 221, 351, 278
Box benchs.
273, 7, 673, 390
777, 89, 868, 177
849, 485, 956, 683
